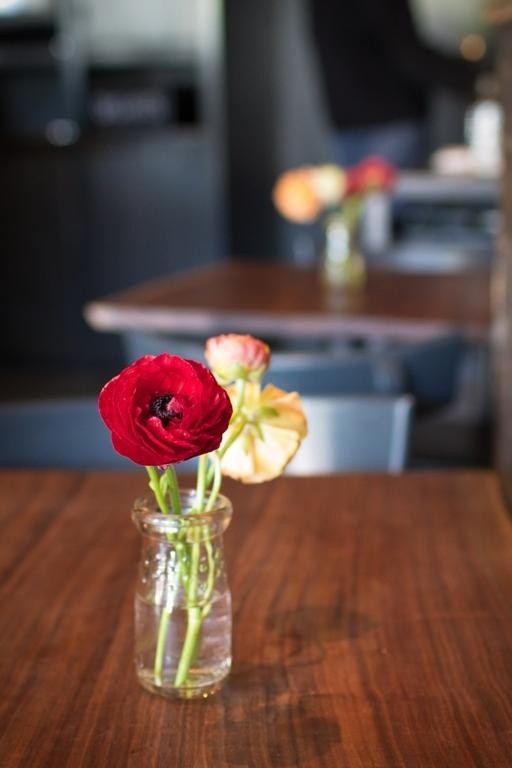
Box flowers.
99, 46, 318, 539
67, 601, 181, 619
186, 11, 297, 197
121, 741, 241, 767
98, 335, 308, 686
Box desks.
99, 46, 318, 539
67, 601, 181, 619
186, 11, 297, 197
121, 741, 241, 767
0, 470, 512, 768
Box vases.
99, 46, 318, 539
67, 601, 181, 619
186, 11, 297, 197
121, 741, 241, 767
131, 491, 232, 701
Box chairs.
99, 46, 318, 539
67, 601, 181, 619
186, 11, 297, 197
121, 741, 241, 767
2, 389, 413, 480
